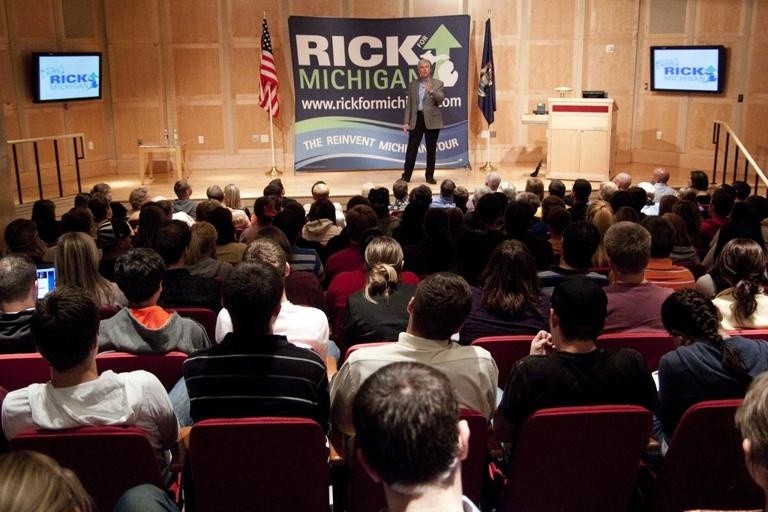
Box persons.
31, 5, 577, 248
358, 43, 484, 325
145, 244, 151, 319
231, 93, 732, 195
5, 218, 48, 268
718, 183, 736, 201
616, 207, 641, 225
568, 181, 592, 222
651, 168, 680, 202
400, 186, 433, 276
0, 253, 44, 353
367, 187, 403, 237
601, 222, 676, 332
660, 289, 768, 446
93, 183, 112, 203
1, 449, 181, 512
733, 181, 752, 202
273, 212, 325, 282
400, 59, 444, 184
263, 185, 306, 247
173, 211, 195, 228
304, 184, 346, 229
687, 172, 712, 205
626, 186, 646, 223
140, 202, 166, 214
2, 289, 180, 491
472, 172, 501, 209
150, 220, 224, 318
533, 195, 565, 241
44, 231, 129, 308
549, 181, 566, 199
295, 199, 342, 247
269, 179, 305, 216
519, 193, 548, 240
341, 237, 419, 355
538, 222, 606, 296
87, 196, 135, 239
493, 277, 662, 482
97, 248, 212, 356
351, 362, 481, 512
468, 192, 508, 236
360, 229, 383, 268
711, 239, 768, 330
476, 202, 553, 271
547, 206, 572, 256
613, 173, 631, 191
697, 190, 730, 232
672, 199, 700, 247
239, 197, 278, 245
216, 238, 339, 362
167, 261, 330, 458
130, 187, 153, 235
662, 213, 701, 266
173, 179, 196, 221
184, 222, 233, 282
458, 240, 552, 347
423, 179, 469, 273
701, 201, 768, 277
256, 226, 329, 319
587, 200, 616, 266
63, 208, 121, 260
224, 184, 251, 222
157, 200, 173, 220
32, 199, 58, 248
658, 195, 679, 216
133, 207, 169, 248
454, 187, 475, 218
525, 179, 544, 220
75, 192, 92, 208
564, 179, 589, 206
598, 181, 619, 200
208, 207, 248, 266
208, 185, 252, 229
311, 181, 326, 195
500, 182, 516, 204
330, 273, 499, 464
679, 183, 700, 207
283, 203, 325, 271
325, 205, 378, 294
706, 223, 744, 276
641, 216, 695, 293
391, 188, 419, 217
608, 190, 630, 214
323, 195, 373, 268
735, 370, 768, 492
196, 200, 222, 222
388, 178, 409, 217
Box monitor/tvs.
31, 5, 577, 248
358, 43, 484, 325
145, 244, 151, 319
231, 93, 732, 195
652, 44, 722, 93
35, 51, 103, 103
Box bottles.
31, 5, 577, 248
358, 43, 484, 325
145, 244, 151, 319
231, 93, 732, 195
172, 129, 179, 146
163, 129, 169, 147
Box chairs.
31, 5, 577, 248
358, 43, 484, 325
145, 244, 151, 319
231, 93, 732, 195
597, 333, 677, 372
98, 307, 116, 320
95, 352, 188, 394
591, 266, 611, 276
166, 308, 217, 344
170, 417, 329, 512
639, 400, 765, 512
0, 353, 53, 392
727, 330, 768, 343
345, 342, 397, 361
488, 406, 653, 512
9, 424, 165, 512
333, 409, 491, 512
471, 336, 535, 391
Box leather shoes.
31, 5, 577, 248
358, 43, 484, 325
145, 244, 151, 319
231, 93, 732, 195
426, 179, 436, 184
399, 178, 410, 182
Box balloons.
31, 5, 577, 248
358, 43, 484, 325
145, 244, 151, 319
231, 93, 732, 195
635, 182, 660, 216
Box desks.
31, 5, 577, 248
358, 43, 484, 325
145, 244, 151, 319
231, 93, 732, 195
138, 141, 188, 185
521, 98, 620, 191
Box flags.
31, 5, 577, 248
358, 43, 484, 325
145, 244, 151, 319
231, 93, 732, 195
476, 8, 497, 126
258, 9, 284, 118
